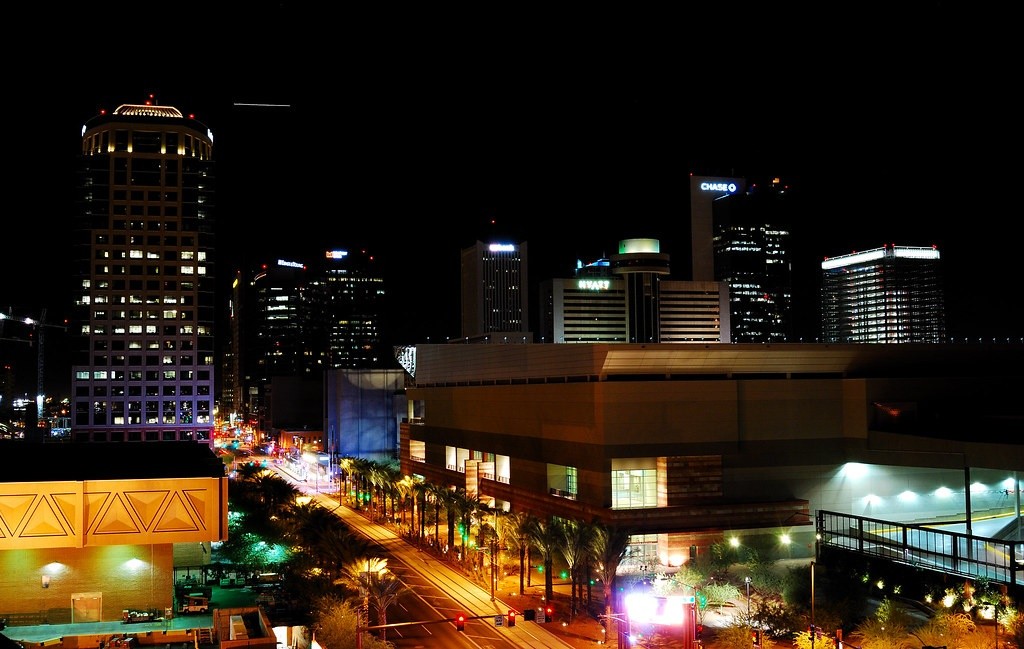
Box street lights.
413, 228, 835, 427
745, 576, 751, 630
661, 576, 699, 625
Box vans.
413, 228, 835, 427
178, 595, 208, 614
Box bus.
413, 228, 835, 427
257, 573, 280, 584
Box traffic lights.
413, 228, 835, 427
696, 624, 703, 640
508, 610, 515, 626
752, 629, 762, 647
833, 638, 839, 649
457, 613, 464, 631
545, 607, 552, 622
807, 626, 814, 640
